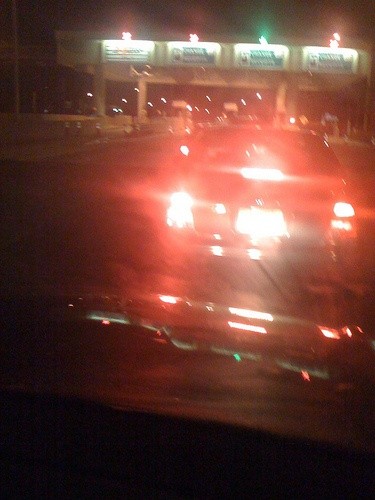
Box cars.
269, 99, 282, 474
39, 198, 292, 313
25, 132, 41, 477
168, 119, 347, 273
